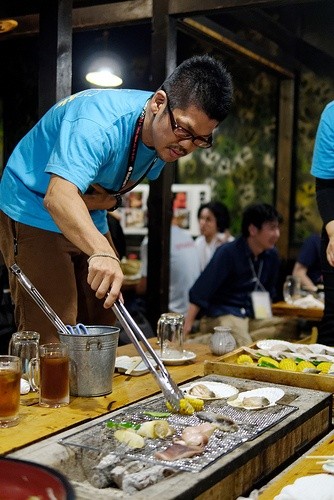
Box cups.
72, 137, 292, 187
157, 313, 185, 359
28, 342, 69, 409
8, 330, 40, 393
0, 355, 22, 429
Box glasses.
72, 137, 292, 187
160, 86, 212, 149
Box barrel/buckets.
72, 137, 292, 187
57, 325, 120, 397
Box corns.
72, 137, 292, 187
279, 358, 298, 372
257, 357, 279, 368
298, 360, 316, 371
166, 397, 205, 415
316, 362, 331, 374
236, 355, 253, 366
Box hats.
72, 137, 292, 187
198, 201, 232, 233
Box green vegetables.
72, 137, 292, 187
106, 420, 141, 431
142, 412, 170, 417
256, 353, 324, 369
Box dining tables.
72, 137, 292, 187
0, 334, 334, 500
272, 296, 324, 320
120, 272, 148, 294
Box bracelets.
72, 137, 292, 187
107, 194, 123, 212
87, 254, 120, 263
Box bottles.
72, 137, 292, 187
283, 276, 301, 304
210, 326, 236, 355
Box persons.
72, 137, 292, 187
292, 231, 325, 293
0, 52, 235, 347
308, 98, 334, 348
195, 201, 235, 271
167, 219, 200, 314
184, 202, 300, 347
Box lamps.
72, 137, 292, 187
82, 32, 126, 88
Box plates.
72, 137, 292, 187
115, 355, 163, 376
153, 349, 196, 366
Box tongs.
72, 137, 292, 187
105, 292, 185, 412
9, 263, 71, 334
